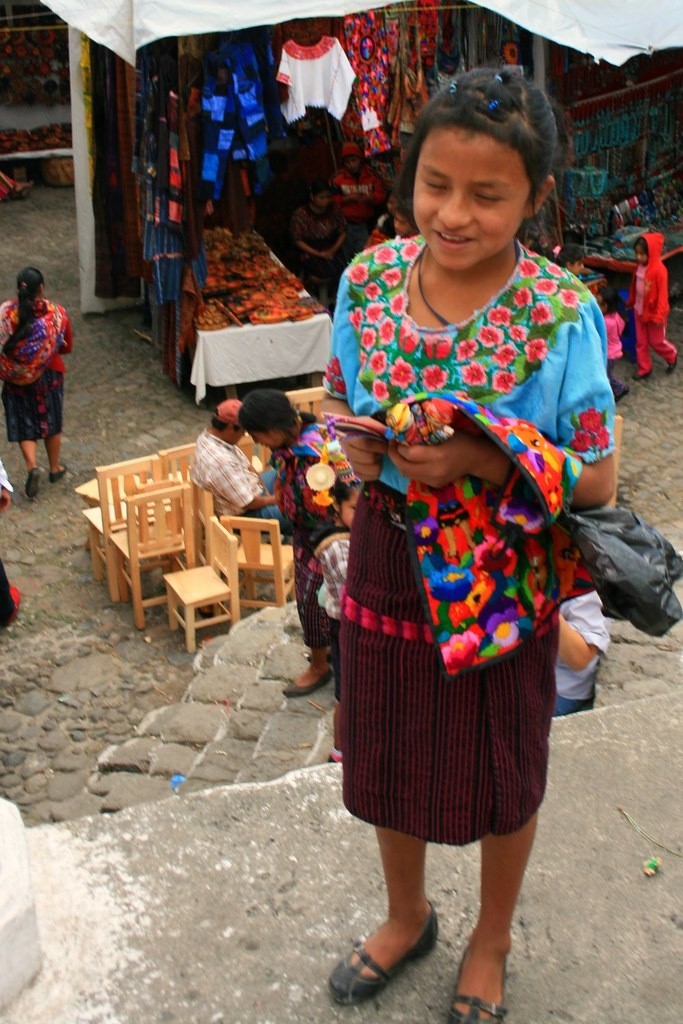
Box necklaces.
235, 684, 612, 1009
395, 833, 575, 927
417, 236, 520, 326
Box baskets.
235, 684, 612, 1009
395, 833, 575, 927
41, 157, 75, 187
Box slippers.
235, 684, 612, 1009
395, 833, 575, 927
10, 180, 34, 199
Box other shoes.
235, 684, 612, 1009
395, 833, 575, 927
307, 651, 333, 663
667, 348, 678, 369
632, 370, 652, 380
49, 462, 67, 483
5, 586, 20, 626
615, 385, 629, 402
328, 898, 438, 1005
446, 948, 509, 1023
25, 468, 40, 499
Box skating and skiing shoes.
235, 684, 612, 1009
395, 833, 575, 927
570, 507, 683, 638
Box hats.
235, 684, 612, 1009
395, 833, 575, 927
341, 142, 362, 157
213, 398, 242, 428
0, 26, 71, 106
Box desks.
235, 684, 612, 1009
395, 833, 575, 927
582, 246, 683, 274
190, 231, 334, 406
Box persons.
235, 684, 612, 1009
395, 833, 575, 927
364, 191, 421, 252
551, 590, 611, 716
551, 243, 629, 405
625, 232, 678, 381
292, 182, 349, 296
0, 266, 73, 498
238, 390, 361, 764
190, 398, 294, 545
319, 62, 616, 1024
0, 458, 21, 629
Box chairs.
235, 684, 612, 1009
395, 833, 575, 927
75, 386, 328, 653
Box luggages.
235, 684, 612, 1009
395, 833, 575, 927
284, 667, 334, 697
